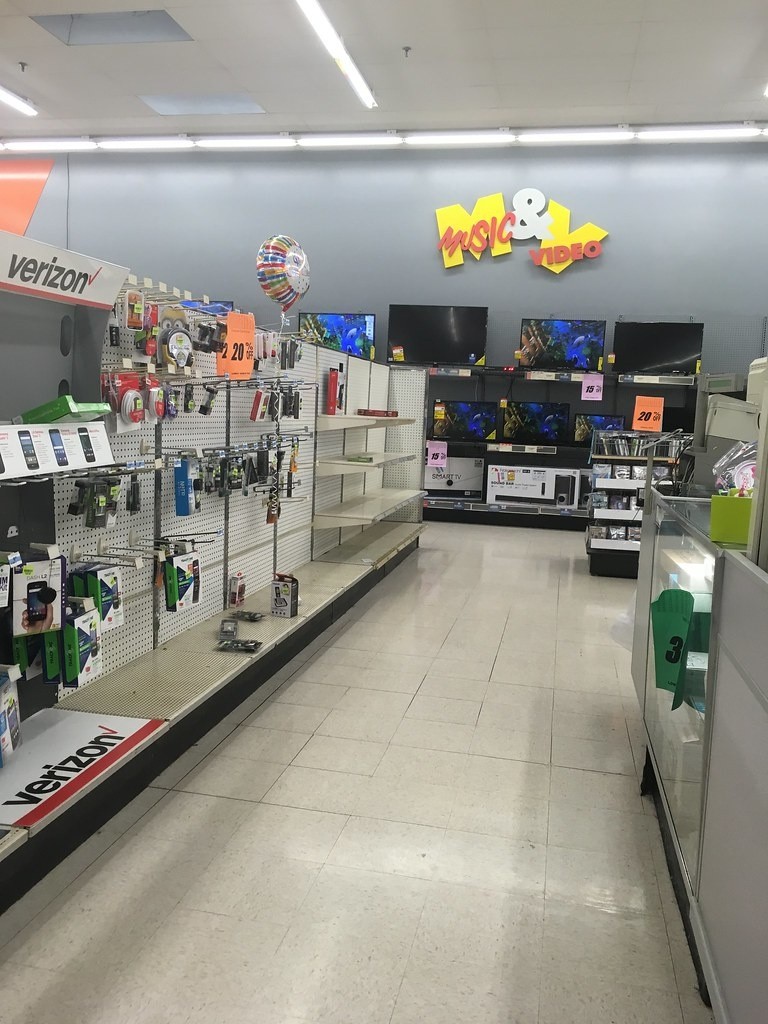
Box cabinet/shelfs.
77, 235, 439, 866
1, 231, 766, 1023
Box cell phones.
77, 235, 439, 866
48, 429, 68, 466
191, 559, 201, 604
27, 581, 47, 620
275, 586, 280, 597
17, 430, 39, 471
111, 577, 119, 609
6, 699, 21, 750
77, 427, 95, 462
194, 489, 201, 509
89, 621, 98, 658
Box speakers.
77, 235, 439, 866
554, 475, 576, 506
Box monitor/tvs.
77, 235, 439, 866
432, 400, 499, 443
612, 321, 704, 377
573, 413, 626, 449
503, 401, 570, 446
386, 303, 489, 368
518, 318, 607, 375
745, 357, 768, 405
298, 312, 376, 363
179, 300, 234, 319
